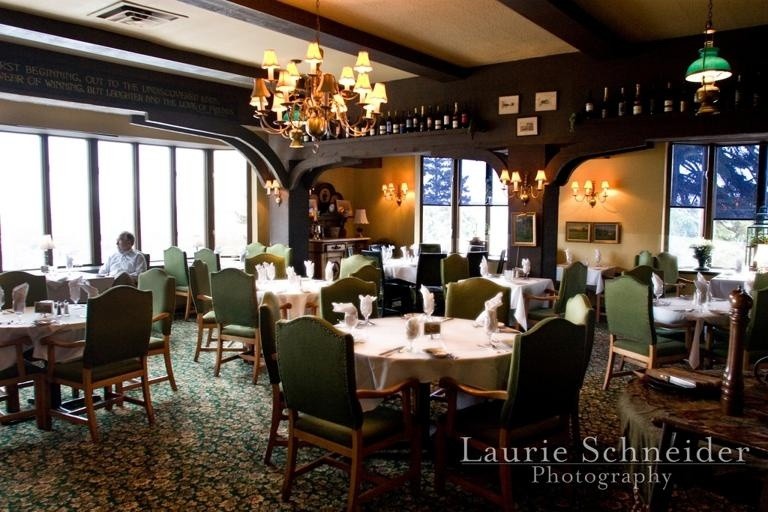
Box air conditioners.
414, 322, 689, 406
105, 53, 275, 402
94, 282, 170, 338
86, 0, 188, 31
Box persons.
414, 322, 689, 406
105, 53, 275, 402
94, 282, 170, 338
99, 231, 146, 279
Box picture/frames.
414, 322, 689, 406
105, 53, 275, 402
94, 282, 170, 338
335, 199, 352, 216
591, 222, 620, 243
534, 91, 557, 111
516, 116, 538, 136
565, 221, 591, 243
510, 211, 538, 248
497, 95, 519, 115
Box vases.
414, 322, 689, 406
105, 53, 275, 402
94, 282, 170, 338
311, 221, 321, 240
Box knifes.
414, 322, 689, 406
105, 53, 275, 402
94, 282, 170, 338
379, 345, 405, 356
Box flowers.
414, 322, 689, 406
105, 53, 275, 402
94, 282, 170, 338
308, 208, 320, 221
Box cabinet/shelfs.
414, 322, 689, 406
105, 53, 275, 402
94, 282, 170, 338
297, 125, 482, 150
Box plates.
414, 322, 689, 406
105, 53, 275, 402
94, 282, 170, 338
424, 348, 450, 359
31, 319, 57, 326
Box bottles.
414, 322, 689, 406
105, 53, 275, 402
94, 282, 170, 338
56, 299, 70, 318
584, 71, 768, 123
301, 99, 469, 143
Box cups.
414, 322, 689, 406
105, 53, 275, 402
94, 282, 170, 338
330, 227, 341, 239
14, 312, 23, 325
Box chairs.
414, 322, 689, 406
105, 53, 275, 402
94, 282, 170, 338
256, 291, 307, 464
0, 335, 52, 430
0, 270, 48, 310
37, 283, 155, 445
428, 315, 587, 512
273, 315, 416, 511
115, 267, 179, 406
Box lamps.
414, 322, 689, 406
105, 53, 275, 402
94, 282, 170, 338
382, 183, 409, 206
498, 167, 548, 207
282, 104, 306, 148
264, 173, 282, 208
571, 179, 609, 208
684, 0, 734, 118
353, 208, 370, 238
249, 1, 390, 154
744, 206, 768, 271
37, 234, 56, 273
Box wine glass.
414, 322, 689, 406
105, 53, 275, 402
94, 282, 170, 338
482, 309, 499, 348
522, 263, 530, 281
422, 295, 434, 322
257, 264, 334, 290
480, 265, 488, 279
342, 312, 358, 343
358, 299, 375, 327
0, 285, 5, 325
311, 225, 322, 240
652, 284, 709, 313
68, 286, 82, 310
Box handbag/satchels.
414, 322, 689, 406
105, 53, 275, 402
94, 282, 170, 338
645, 365, 722, 396
